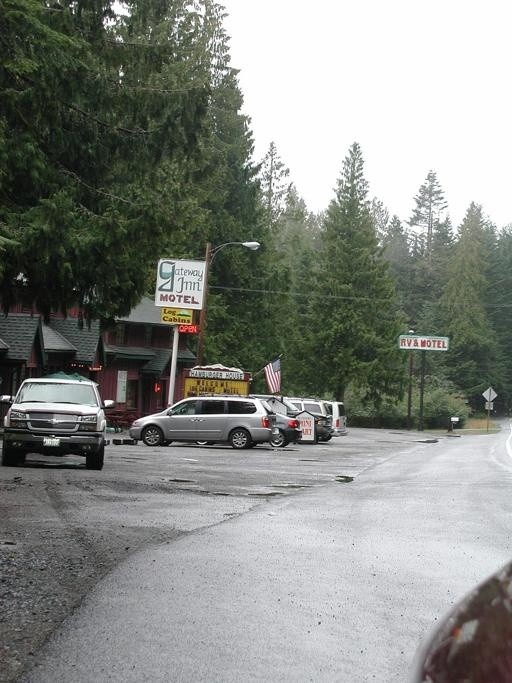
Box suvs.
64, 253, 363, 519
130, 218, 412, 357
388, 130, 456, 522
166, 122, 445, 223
0, 376, 104, 469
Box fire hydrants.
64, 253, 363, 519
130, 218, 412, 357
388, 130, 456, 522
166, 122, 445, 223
447, 416, 456, 432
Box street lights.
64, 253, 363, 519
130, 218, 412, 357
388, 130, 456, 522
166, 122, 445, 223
195, 239, 260, 368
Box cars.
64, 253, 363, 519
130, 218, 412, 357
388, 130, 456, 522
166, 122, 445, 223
130, 391, 349, 450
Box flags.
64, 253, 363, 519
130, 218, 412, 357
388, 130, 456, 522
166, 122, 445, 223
263, 357, 281, 394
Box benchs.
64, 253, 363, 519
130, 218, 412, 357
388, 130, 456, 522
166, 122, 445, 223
105, 410, 139, 433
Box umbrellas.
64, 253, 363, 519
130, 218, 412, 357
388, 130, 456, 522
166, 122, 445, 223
68, 372, 100, 386
40, 371, 72, 380
